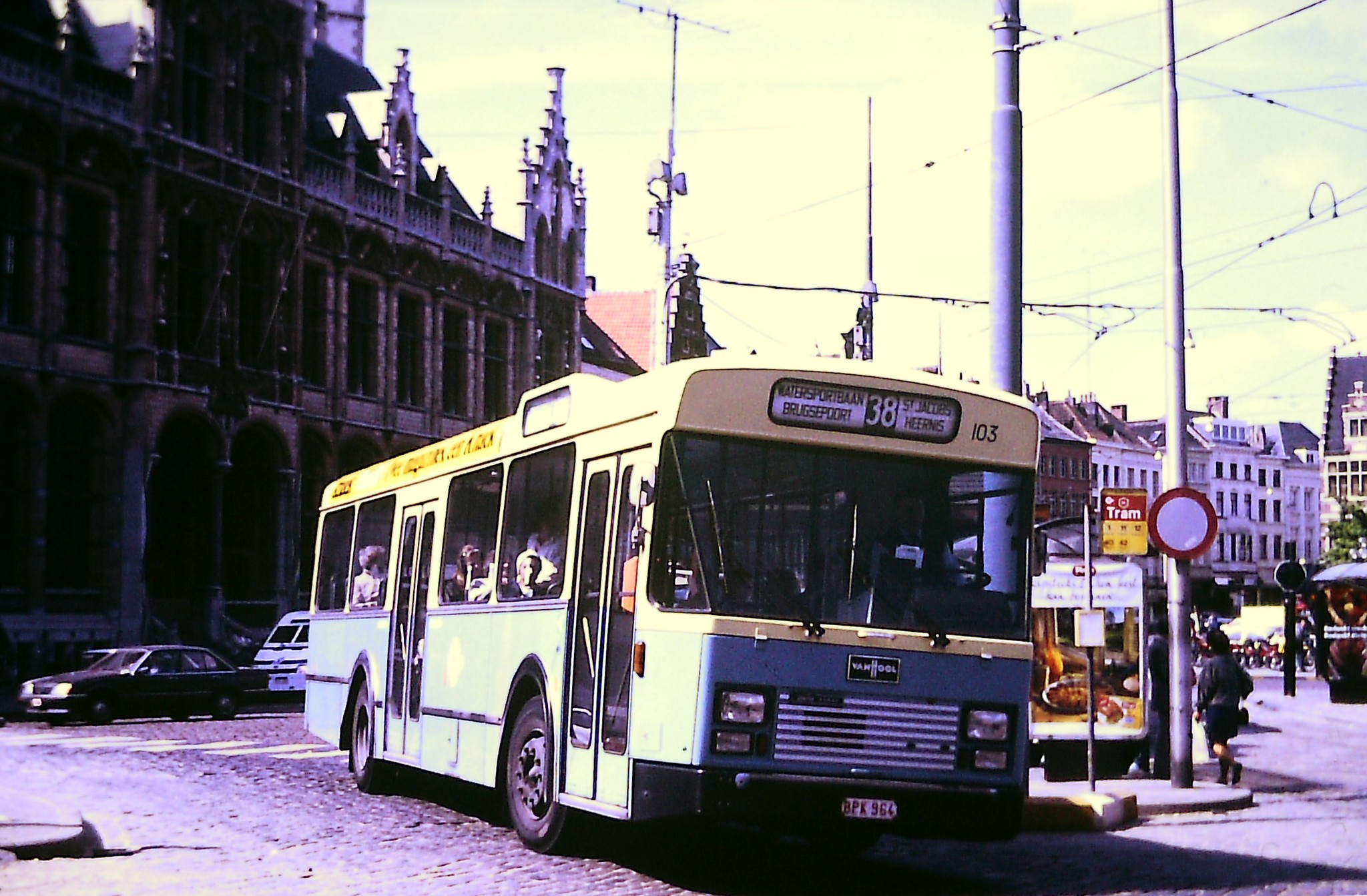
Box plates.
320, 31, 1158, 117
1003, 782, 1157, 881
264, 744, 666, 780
1042, 678, 1116, 710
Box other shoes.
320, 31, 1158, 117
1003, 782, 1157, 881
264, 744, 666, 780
1232, 763, 1243, 785
1216, 777, 1228, 785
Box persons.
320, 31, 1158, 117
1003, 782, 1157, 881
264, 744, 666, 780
504, 549, 547, 597
1194, 629, 1245, 784
1148, 618, 1170, 778
527, 516, 565, 566
1208, 611, 1218, 630
443, 545, 495, 601
869, 490, 967, 586
1295, 616, 1312, 672
352, 545, 387, 607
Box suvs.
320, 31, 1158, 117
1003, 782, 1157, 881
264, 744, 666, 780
253, 611, 313, 710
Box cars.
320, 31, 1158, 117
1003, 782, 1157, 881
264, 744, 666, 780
20, 645, 245, 725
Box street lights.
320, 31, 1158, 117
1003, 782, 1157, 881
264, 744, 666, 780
644, 158, 688, 364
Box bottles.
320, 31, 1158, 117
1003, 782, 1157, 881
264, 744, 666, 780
1121, 702, 1137, 727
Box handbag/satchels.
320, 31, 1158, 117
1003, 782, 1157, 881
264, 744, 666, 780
1239, 707, 1249, 726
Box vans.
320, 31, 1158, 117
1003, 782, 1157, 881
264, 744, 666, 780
1199, 604, 1287, 649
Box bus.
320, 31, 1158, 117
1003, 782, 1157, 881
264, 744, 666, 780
302, 352, 1052, 857
